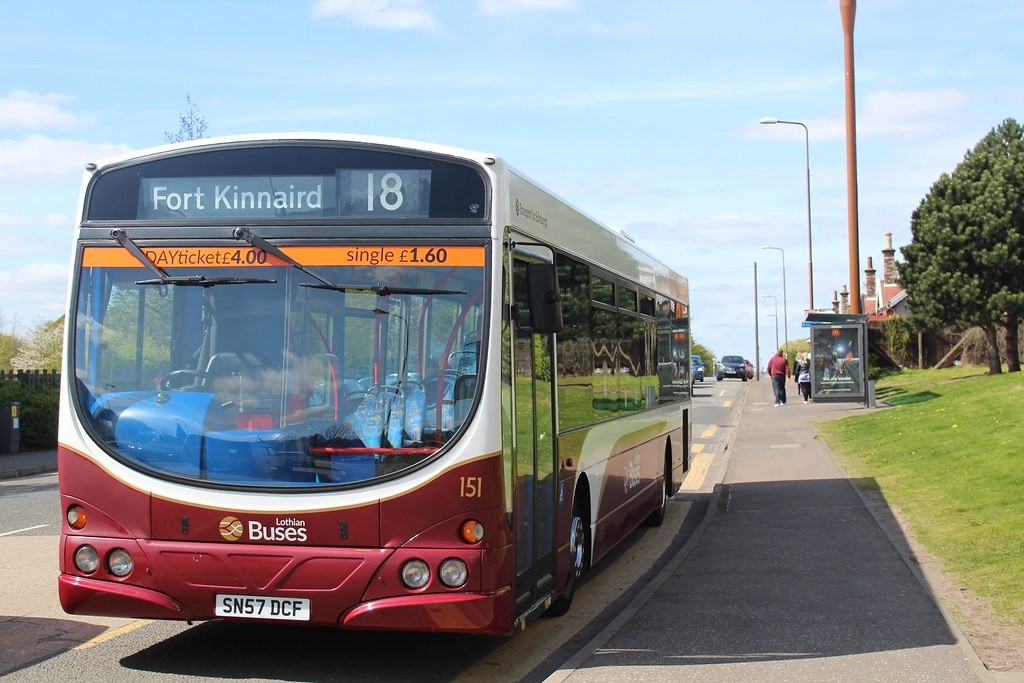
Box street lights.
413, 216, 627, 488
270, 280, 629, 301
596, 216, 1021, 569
763, 247, 788, 363
759, 118, 814, 311
761, 296, 778, 353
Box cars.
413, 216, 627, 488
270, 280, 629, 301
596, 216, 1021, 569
717, 355, 754, 381
691, 355, 705, 382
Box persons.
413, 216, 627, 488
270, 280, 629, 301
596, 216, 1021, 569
793, 352, 812, 404
767, 349, 791, 407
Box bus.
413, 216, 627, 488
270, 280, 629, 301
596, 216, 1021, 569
57, 132, 694, 639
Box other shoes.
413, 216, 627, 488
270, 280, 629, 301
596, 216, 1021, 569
781, 401, 786, 405
803, 401, 807, 404
772, 404, 779, 407
809, 399, 812, 402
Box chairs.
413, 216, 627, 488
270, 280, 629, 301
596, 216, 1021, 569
285, 331, 478, 451
201, 351, 264, 398
